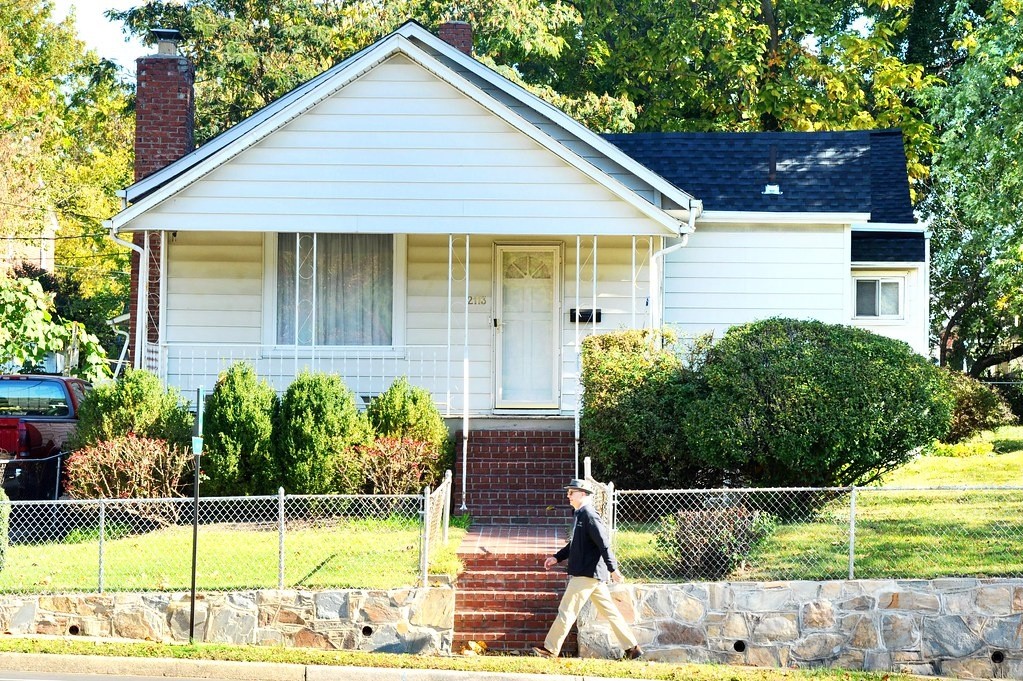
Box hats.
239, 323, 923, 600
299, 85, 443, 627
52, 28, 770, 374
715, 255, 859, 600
563, 479, 594, 494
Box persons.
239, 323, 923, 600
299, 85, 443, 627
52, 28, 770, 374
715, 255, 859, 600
532, 478, 644, 661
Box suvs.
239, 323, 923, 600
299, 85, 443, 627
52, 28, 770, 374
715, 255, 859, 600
0, 374, 95, 456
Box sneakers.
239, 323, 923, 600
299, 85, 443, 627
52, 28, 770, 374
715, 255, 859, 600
532, 647, 557, 658
621, 646, 644, 660
